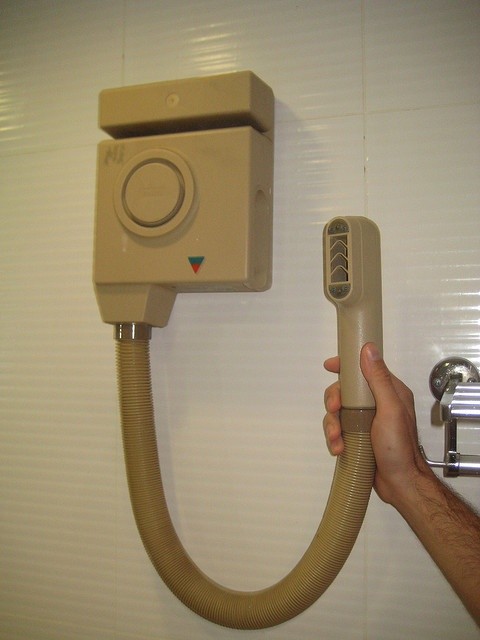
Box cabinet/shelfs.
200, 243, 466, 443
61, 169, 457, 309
417, 355, 480, 480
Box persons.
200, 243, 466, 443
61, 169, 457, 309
323, 342, 480, 629
